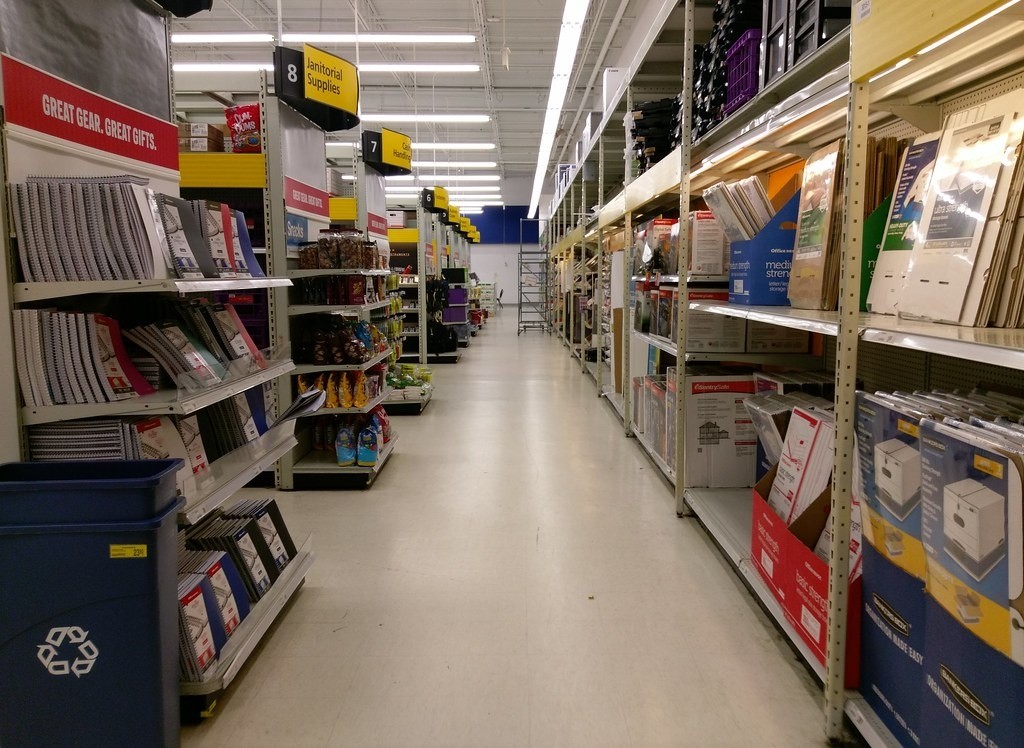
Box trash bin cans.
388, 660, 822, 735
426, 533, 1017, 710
0, 457, 186, 748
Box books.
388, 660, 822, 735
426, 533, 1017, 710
185, 499, 299, 604
12, 293, 269, 407
178, 527, 256, 683
24, 420, 134, 462
133, 377, 277, 506
268, 389, 327, 431
10, 173, 266, 283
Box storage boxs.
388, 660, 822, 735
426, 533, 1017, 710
178, 137, 225, 152
177, 122, 224, 145
758, 1, 853, 91
728, 187, 802, 306
477, 282, 498, 318
923, 590, 1022, 747
857, 534, 927, 747
723, 28, 758, 116
784, 481, 859, 690
632, 210, 866, 489
749, 461, 789, 609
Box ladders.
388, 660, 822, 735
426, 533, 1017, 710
517, 218, 551, 336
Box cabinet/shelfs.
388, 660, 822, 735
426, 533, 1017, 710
943, 477, 1006, 581
674, 1, 871, 746
177, 69, 400, 492
0, 122, 316, 724
875, 438, 922, 522
532, 0, 694, 518
325, 145, 487, 417
819, 1, 1022, 748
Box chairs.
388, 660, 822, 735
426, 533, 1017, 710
496, 289, 504, 309
